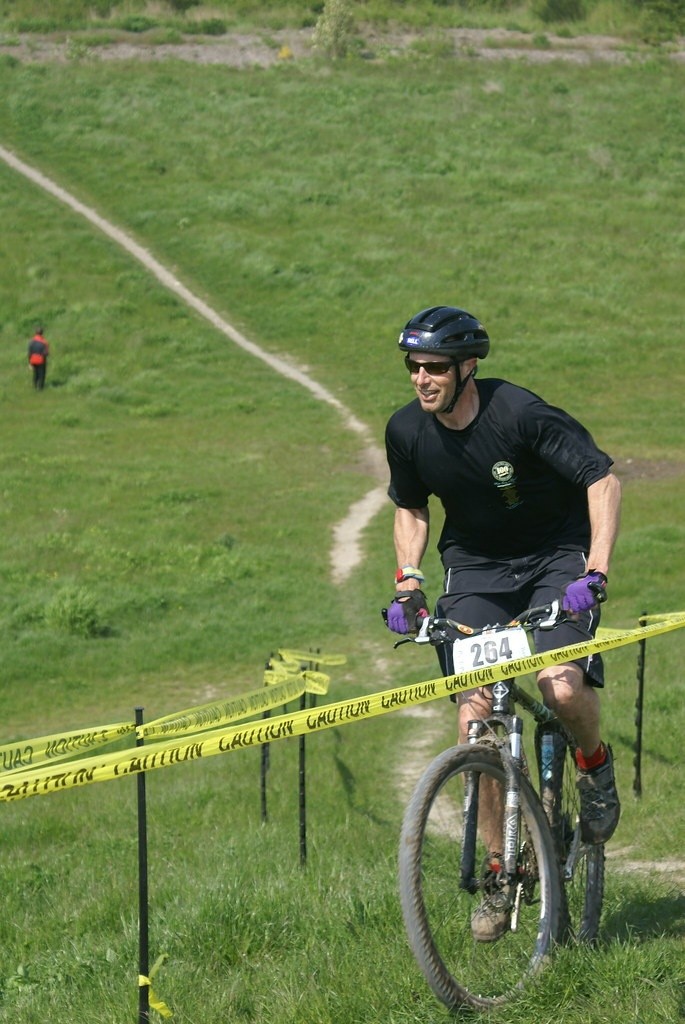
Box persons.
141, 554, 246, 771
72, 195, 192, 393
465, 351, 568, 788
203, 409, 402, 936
28, 327, 49, 389
384, 305, 621, 942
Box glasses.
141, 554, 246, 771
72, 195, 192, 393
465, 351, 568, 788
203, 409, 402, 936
405, 353, 454, 375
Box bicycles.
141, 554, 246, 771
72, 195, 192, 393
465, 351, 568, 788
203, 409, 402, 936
379, 583, 607, 1018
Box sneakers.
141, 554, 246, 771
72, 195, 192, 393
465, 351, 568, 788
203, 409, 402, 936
470, 884, 517, 943
574, 739, 621, 846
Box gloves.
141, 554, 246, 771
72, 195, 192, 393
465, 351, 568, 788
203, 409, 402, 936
387, 589, 430, 635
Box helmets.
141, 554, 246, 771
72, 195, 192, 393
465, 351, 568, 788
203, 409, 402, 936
398, 305, 490, 362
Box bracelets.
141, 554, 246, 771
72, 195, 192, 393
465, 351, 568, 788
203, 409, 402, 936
395, 563, 425, 585
